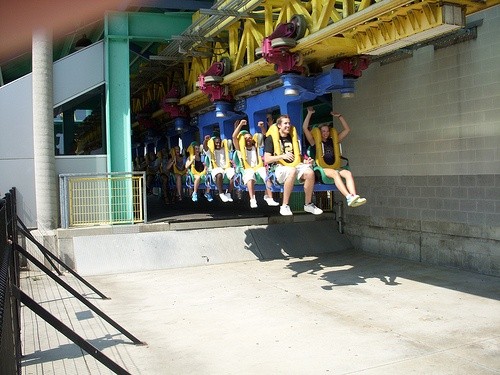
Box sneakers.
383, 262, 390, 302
225, 189, 233, 202
249, 195, 257, 208
204, 191, 214, 202
280, 204, 293, 216
264, 195, 279, 206
191, 191, 198, 201
219, 193, 228, 202
303, 203, 324, 215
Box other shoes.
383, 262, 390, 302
236, 189, 243, 200
351, 198, 367, 207
163, 193, 170, 205
178, 194, 183, 201
170, 191, 176, 205
347, 194, 360, 206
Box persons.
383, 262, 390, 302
303, 106, 367, 207
231, 119, 279, 208
167, 146, 195, 200
202, 134, 235, 202
263, 114, 323, 215
132, 151, 172, 197
186, 144, 213, 202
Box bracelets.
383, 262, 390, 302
338, 114, 342, 118
